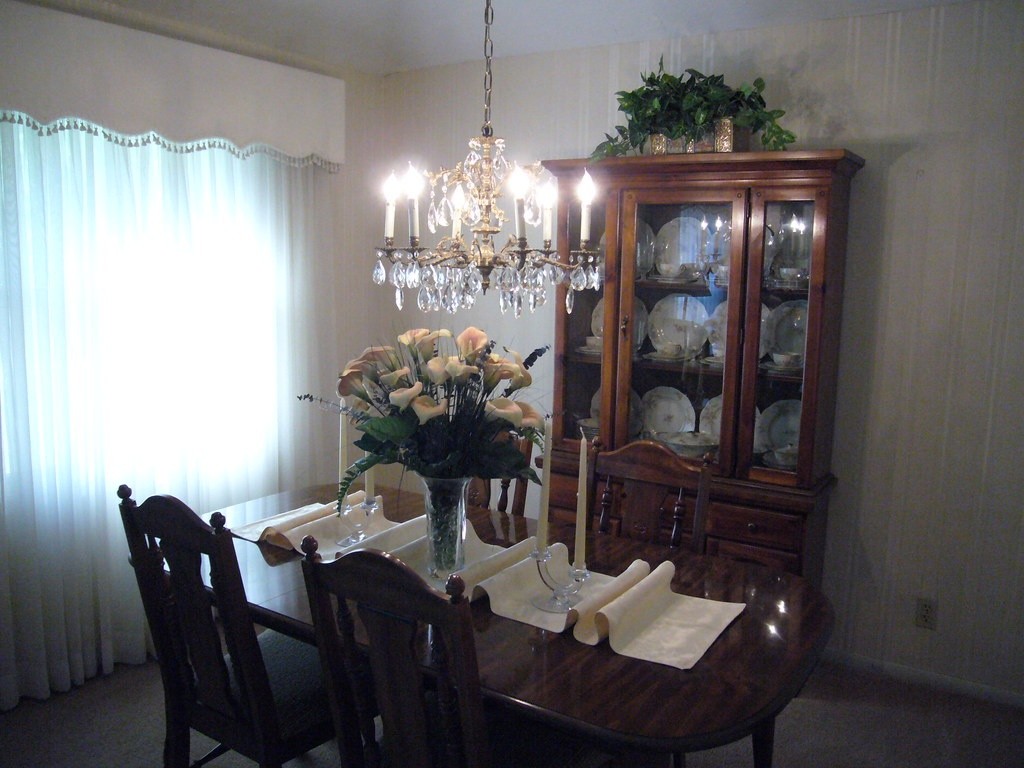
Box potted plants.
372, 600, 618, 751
587, 55, 795, 160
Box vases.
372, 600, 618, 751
416, 474, 474, 580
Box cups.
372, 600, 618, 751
774, 443, 797, 465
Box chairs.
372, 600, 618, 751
596, 433, 713, 551
301, 533, 672, 767
117, 485, 383, 767
469, 429, 532, 516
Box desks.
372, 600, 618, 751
179, 482, 836, 768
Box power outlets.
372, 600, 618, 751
914, 597, 938, 631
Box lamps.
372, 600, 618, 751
373, 1, 606, 316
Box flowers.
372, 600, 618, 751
300, 326, 545, 563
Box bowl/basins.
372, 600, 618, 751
657, 431, 719, 458
585, 336, 603, 349
719, 265, 729, 277
712, 342, 725, 357
773, 351, 801, 366
779, 267, 805, 279
755, 445, 771, 461
661, 263, 697, 274
657, 341, 681, 355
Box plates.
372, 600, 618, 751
574, 293, 809, 376
597, 216, 810, 289
575, 419, 599, 441
642, 386, 802, 469
590, 383, 641, 437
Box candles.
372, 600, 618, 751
536, 416, 553, 553
365, 450, 374, 505
339, 395, 348, 491
574, 426, 588, 573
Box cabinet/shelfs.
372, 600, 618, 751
547, 148, 867, 583
